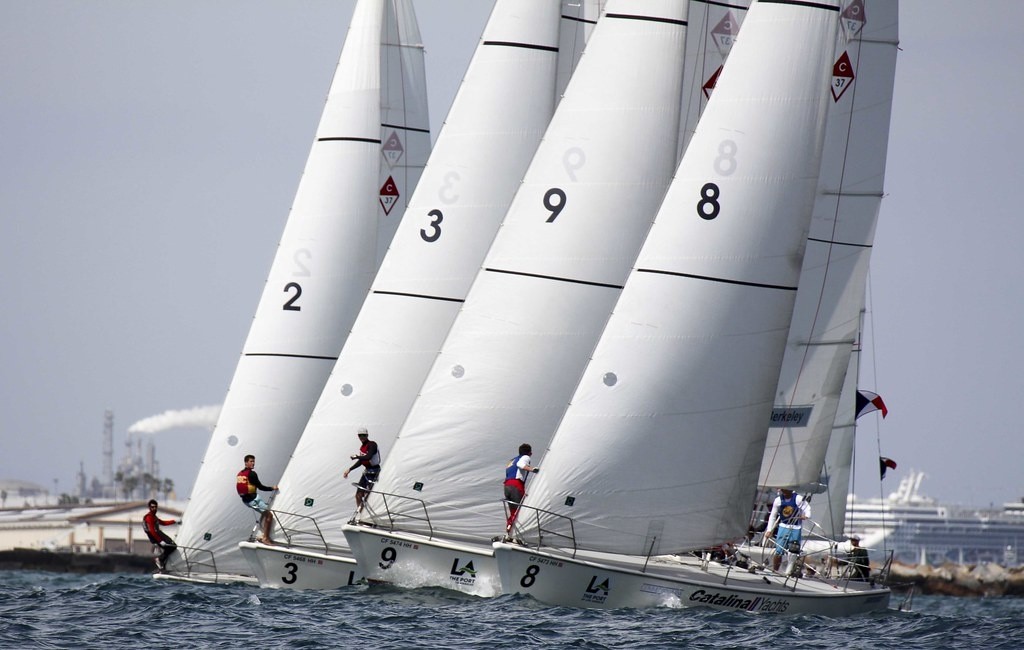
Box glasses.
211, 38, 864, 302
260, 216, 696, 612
150, 506, 157, 508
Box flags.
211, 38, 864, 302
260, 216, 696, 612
857, 390, 888, 420
880, 457, 897, 481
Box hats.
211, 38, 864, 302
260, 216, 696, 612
850, 535, 860, 542
357, 428, 368, 434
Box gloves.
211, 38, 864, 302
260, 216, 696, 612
160, 541, 167, 546
532, 467, 539, 473
175, 521, 182, 524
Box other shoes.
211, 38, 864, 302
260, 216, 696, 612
159, 570, 168, 574
155, 558, 164, 569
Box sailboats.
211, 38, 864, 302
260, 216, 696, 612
153, 1, 898, 623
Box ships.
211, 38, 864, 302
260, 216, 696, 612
845, 470, 1024, 564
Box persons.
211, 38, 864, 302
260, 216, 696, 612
344, 428, 380, 513
694, 541, 734, 562
143, 499, 181, 574
756, 506, 780, 536
841, 536, 869, 581
504, 444, 539, 541
236, 455, 278, 545
765, 489, 812, 577
